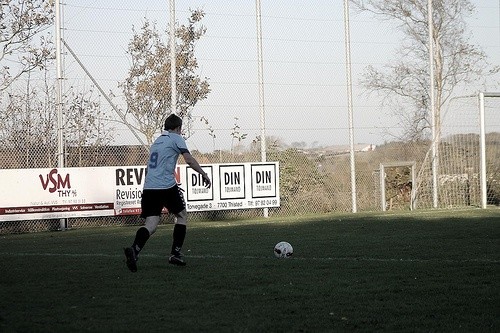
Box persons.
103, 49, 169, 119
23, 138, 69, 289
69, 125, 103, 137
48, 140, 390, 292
124, 114, 211, 272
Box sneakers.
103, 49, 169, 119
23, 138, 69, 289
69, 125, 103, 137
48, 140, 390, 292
168, 252, 187, 267
124, 248, 139, 272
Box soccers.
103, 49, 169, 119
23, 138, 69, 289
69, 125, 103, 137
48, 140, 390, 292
273, 241, 293, 259
